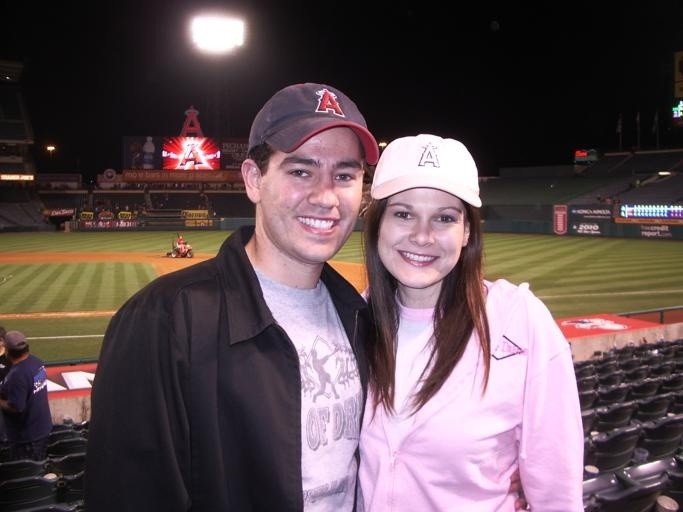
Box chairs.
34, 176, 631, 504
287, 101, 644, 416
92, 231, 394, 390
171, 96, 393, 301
0, 339, 683, 512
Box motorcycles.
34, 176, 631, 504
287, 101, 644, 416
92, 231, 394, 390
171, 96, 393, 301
172, 243, 193, 258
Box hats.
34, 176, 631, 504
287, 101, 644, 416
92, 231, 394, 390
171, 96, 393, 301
370, 132, 483, 209
4, 330, 29, 350
246, 81, 378, 168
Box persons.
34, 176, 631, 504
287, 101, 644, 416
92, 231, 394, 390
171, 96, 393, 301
176, 232, 185, 246
0, 330, 52, 461
82, 83, 377, 512
81, 192, 207, 213
0, 325, 12, 442
354, 133, 590, 512
